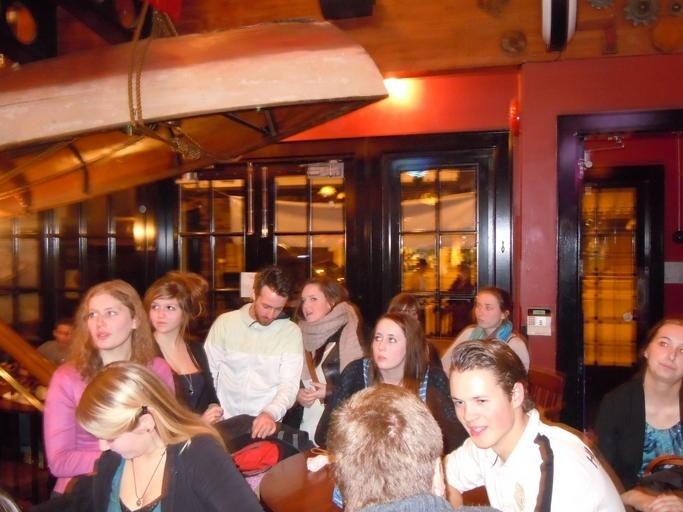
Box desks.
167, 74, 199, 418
0, 363, 45, 466
260, 447, 343, 512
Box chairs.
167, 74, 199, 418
528, 369, 567, 422
644, 456, 683, 475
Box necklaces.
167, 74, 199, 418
131, 448, 166, 505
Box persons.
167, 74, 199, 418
76, 361, 264, 511
143, 271, 223, 425
201, 267, 303, 440
594, 318, 683, 512
386, 292, 439, 360
42, 279, 175, 511
294, 274, 366, 448
315, 314, 468, 451
19, 320, 72, 470
442, 341, 626, 512
441, 287, 529, 372
324, 384, 453, 512
